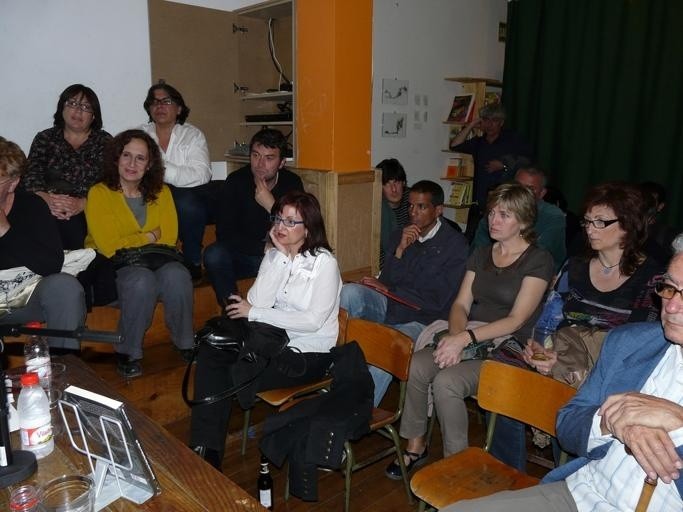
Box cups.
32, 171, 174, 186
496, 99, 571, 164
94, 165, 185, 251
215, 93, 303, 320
26, 363, 65, 410
531, 325, 558, 362
38, 473, 93, 510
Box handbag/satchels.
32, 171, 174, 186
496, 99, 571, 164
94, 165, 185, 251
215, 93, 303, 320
195, 314, 289, 364
116, 244, 185, 270
433, 328, 492, 360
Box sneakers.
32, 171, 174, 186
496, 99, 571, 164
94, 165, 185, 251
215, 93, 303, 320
174, 344, 195, 363
115, 353, 142, 378
190, 265, 202, 286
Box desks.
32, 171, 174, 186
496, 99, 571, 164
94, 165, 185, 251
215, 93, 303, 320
1, 347, 273, 512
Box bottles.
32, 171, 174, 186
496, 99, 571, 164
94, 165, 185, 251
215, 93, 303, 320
256, 454, 272, 509
24, 322, 55, 404
18, 373, 54, 459
8, 486, 40, 510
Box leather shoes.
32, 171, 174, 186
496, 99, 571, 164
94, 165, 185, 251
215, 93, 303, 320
386, 445, 430, 480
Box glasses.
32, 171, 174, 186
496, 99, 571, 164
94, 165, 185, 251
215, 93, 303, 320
579, 219, 618, 228
270, 216, 304, 227
653, 282, 683, 299
64, 100, 94, 113
151, 98, 176, 105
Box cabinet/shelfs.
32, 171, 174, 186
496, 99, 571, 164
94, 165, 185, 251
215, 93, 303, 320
147, 0, 374, 174
441, 76, 503, 224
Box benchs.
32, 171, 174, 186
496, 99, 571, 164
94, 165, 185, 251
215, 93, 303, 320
0, 221, 258, 431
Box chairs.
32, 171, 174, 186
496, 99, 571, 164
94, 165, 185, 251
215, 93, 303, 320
236, 307, 347, 462
276, 313, 422, 512
407, 355, 584, 512
